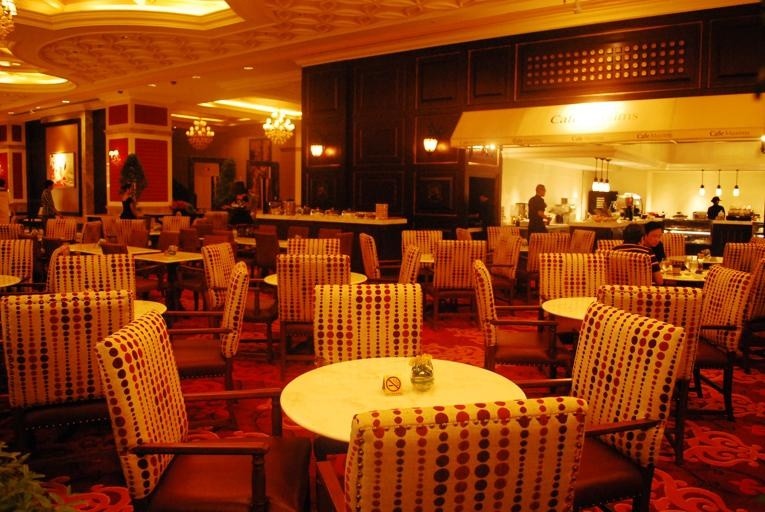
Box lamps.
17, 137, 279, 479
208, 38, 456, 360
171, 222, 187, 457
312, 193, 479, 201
716, 169, 722, 196
311, 142, 323, 161
424, 132, 438, 158
185, 119, 216, 151
734, 169, 739, 196
592, 157, 612, 192
699, 169, 705, 196
263, 110, 294, 146
109, 150, 121, 163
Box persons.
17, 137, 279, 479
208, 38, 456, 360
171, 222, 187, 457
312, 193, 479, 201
643, 221, 666, 262
0, 178, 10, 224
40, 180, 64, 219
611, 223, 663, 285
708, 196, 725, 220
620, 197, 640, 217
527, 184, 550, 245
120, 184, 145, 219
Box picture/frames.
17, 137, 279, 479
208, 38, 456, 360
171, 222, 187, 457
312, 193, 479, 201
47, 152, 76, 189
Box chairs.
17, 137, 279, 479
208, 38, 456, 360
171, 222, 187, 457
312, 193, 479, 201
314, 396, 588, 512
95, 308, 311, 512
1, 291, 134, 476
512, 302, 685, 512
0, 211, 764, 423
597, 285, 702, 464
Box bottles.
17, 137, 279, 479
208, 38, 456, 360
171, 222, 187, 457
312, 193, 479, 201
697, 254, 704, 269
286, 198, 295, 216
